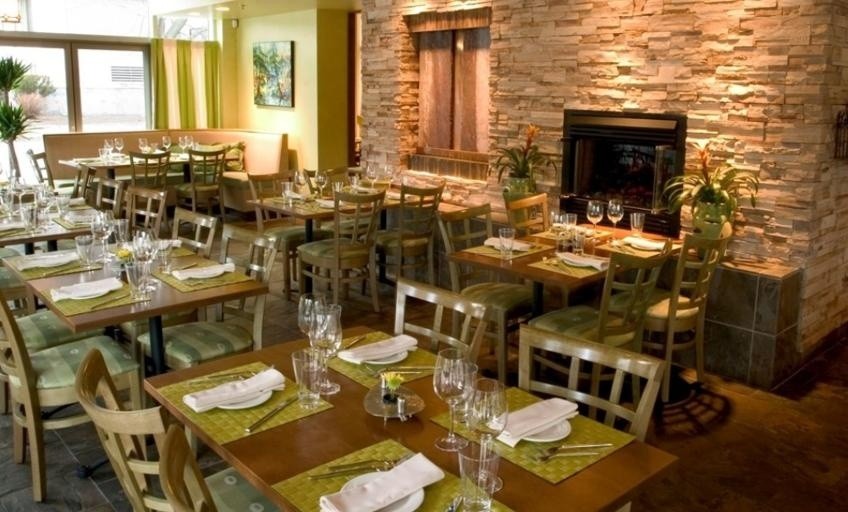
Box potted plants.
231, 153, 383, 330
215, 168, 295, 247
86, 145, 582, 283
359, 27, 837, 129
655, 135, 760, 264
0, 55, 38, 203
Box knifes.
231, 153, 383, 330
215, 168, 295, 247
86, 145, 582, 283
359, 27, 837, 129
332, 336, 364, 357
245, 392, 303, 435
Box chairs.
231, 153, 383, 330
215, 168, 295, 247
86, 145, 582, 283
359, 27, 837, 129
0, 127, 731, 511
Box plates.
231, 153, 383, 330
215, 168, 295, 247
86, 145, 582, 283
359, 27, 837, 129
192, 271, 225, 279
562, 258, 591, 268
521, 415, 573, 444
338, 472, 424, 512
359, 344, 409, 364
630, 243, 656, 252
218, 389, 275, 410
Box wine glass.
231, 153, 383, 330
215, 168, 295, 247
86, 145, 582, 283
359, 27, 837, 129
465, 379, 508, 450
433, 349, 468, 449
310, 304, 340, 395
98, 133, 198, 164
1, 169, 71, 238
299, 293, 326, 356
550, 197, 624, 257
76, 207, 173, 303
284, 163, 395, 209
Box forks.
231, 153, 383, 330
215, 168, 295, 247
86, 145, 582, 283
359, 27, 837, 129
362, 362, 441, 376
541, 255, 574, 276
527, 441, 614, 465
189, 364, 277, 386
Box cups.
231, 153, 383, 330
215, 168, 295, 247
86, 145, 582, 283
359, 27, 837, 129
497, 228, 516, 256
456, 438, 502, 511
291, 349, 324, 411
629, 213, 645, 238
